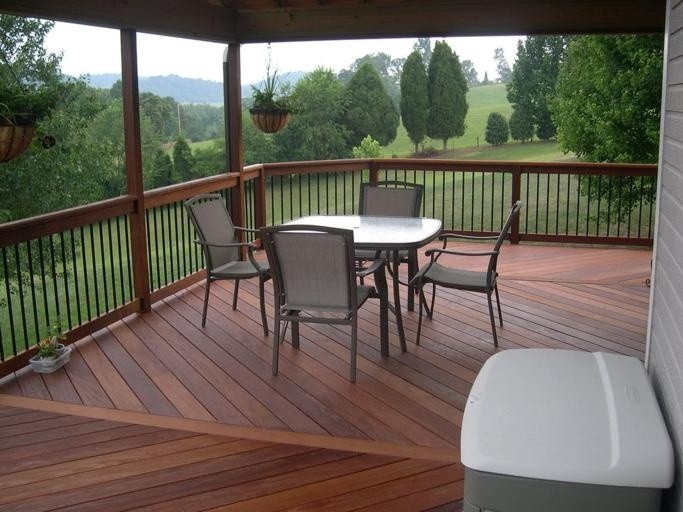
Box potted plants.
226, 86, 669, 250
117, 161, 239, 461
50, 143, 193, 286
33, 316, 70, 368
242, 64, 307, 133
0, 49, 58, 165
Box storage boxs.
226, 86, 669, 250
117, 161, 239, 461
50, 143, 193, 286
458, 349, 677, 512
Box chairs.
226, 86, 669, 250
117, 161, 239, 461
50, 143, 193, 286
409, 200, 523, 347
258, 223, 379, 384
184, 194, 268, 336
354, 180, 424, 301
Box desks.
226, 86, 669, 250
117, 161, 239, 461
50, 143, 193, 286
277, 215, 441, 359
